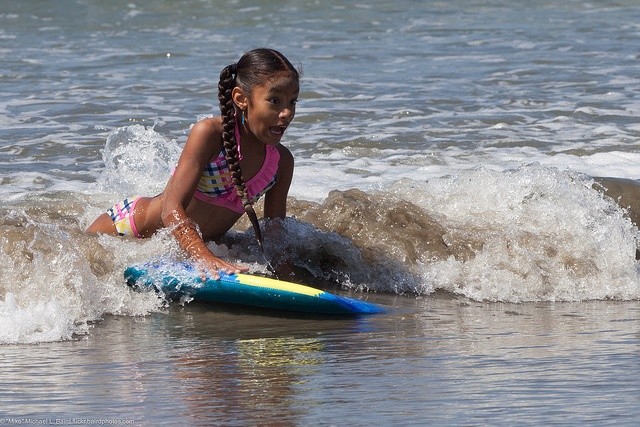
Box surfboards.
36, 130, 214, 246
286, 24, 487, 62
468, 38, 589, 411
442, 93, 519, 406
123, 263, 389, 316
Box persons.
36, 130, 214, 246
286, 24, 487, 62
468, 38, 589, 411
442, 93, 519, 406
85, 48, 300, 285
